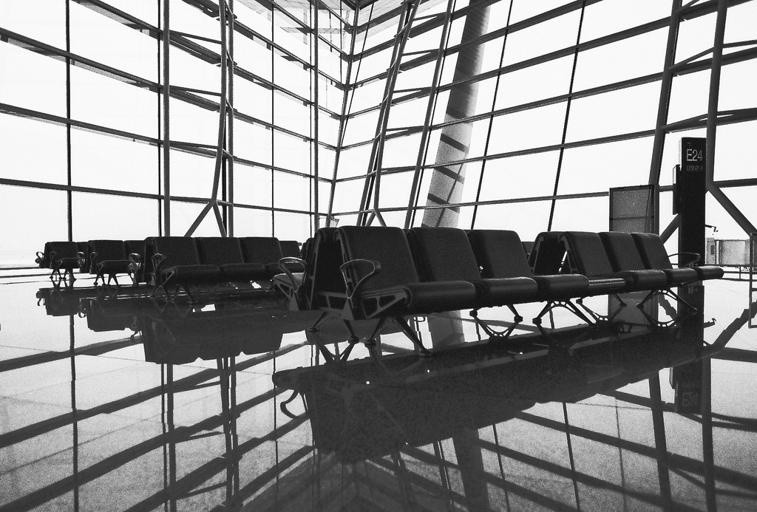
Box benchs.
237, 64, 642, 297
37, 237, 299, 305
272, 226, 723, 348
